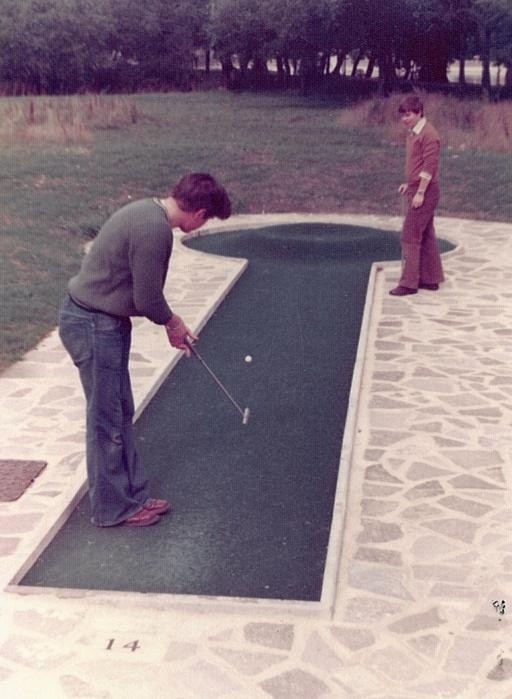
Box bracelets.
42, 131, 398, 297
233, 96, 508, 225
415, 190, 425, 196
168, 319, 184, 331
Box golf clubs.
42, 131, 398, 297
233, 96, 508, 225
184, 337, 250, 425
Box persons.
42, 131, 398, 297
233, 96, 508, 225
57, 172, 233, 529
388, 95, 446, 296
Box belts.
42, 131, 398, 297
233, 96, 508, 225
68, 293, 105, 312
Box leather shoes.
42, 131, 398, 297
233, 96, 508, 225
122, 498, 169, 526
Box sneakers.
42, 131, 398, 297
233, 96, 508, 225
419, 283, 438, 290
390, 286, 418, 295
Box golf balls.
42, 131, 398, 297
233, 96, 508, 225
245, 355, 252, 362
